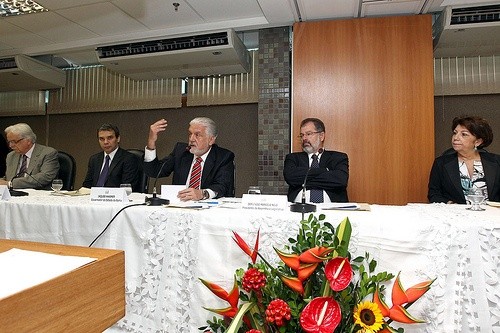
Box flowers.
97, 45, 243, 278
197, 218, 435, 333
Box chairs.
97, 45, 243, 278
125, 149, 152, 193
50, 151, 77, 191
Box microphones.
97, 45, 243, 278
145, 146, 192, 205
9, 171, 45, 196
290, 148, 324, 212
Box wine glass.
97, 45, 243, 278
51, 180, 64, 196
465, 188, 486, 211
120, 184, 133, 202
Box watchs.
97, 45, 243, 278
199, 189, 208, 200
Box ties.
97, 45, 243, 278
18, 155, 27, 177
189, 157, 203, 190
96, 155, 110, 187
310, 154, 323, 203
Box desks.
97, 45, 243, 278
0, 185, 500, 333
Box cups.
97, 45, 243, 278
248, 186, 261, 195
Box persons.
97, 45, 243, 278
427, 114, 500, 204
0, 122, 60, 189
143, 117, 235, 202
80, 122, 142, 193
283, 118, 350, 204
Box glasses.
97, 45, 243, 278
6, 138, 23, 145
298, 132, 321, 138
